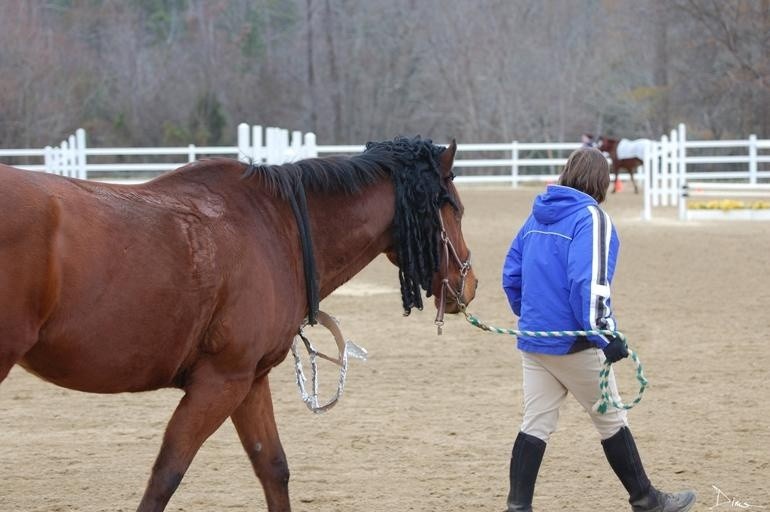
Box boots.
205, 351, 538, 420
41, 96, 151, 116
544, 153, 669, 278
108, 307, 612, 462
601, 427, 696, 511
502, 431, 546, 512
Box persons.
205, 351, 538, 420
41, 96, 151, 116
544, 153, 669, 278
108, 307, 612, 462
502, 144, 697, 512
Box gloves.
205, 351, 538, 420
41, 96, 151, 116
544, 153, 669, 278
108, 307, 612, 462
604, 337, 628, 363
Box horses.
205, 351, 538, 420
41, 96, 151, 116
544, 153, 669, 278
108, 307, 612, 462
0, 132, 479, 512
593, 136, 654, 194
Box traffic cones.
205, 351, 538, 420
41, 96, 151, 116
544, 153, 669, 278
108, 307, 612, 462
613, 175, 623, 193
